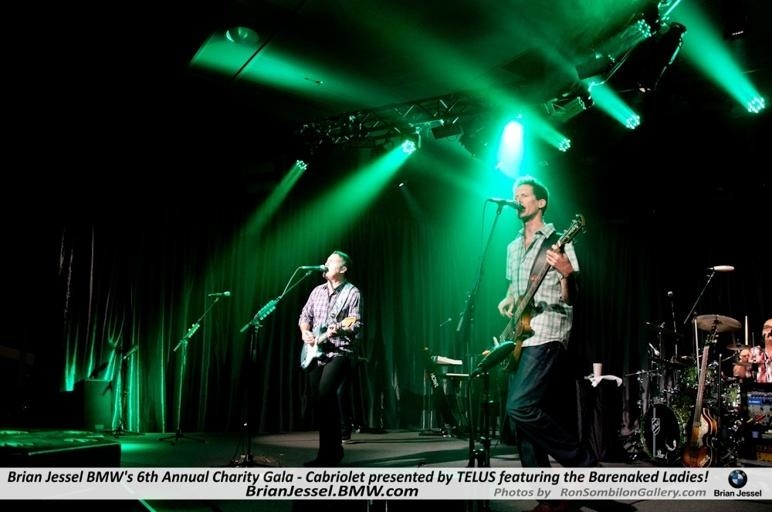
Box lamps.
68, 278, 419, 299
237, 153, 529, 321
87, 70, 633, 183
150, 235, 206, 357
292, 89, 487, 172
502, 0, 673, 130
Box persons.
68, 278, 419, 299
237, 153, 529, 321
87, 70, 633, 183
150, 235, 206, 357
298, 250, 365, 468
731, 319, 772, 383
497, 179, 601, 468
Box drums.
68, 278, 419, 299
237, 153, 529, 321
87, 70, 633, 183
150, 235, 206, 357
640, 403, 690, 463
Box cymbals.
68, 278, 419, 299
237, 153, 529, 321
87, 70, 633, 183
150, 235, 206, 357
691, 314, 742, 332
727, 344, 751, 350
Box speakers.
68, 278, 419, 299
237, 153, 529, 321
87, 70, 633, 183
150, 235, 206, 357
26, 443, 121, 467
76, 378, 112, 431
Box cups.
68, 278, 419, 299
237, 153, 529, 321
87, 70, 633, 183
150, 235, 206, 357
592, 362, 602, 378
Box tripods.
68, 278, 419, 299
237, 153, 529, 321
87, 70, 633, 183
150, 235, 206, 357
159, 354, 205, 444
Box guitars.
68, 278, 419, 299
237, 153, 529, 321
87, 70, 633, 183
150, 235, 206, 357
681, 326, 719, 467
493, 213, 587, 373
300, 316, 357, 369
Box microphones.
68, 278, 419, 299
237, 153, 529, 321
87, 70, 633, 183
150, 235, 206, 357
707, 265, 735, 272
300, 265, 328, 272
208, 290, 232, 298
489, 197, 523, 212
468, 341, 518, 378
123, 345, 139, 361
668, 291, 674, 304
